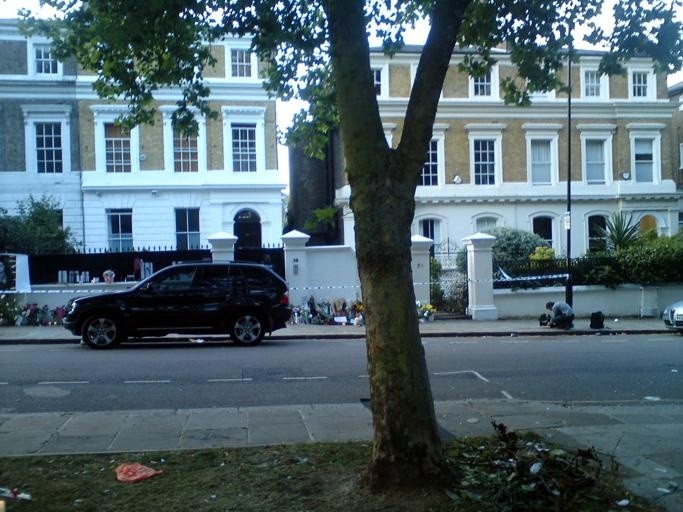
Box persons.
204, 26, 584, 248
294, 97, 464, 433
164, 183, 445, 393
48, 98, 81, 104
544, 301, 575, 330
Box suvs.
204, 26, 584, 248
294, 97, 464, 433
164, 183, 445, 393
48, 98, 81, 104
57, 258, 294, 351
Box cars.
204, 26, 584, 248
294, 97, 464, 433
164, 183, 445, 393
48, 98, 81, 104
661, 299, 683, 337
